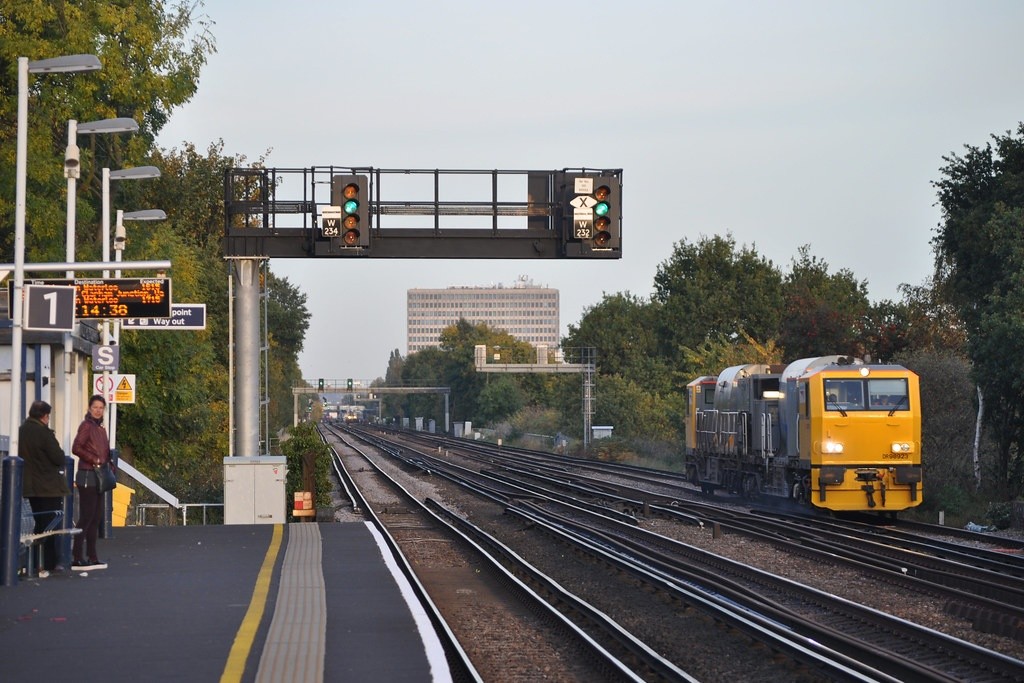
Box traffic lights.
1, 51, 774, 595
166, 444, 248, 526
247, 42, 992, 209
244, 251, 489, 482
347, 379, 353, 391
342, 182, 362, 246
595, 185, 612, 248
308, 403, 312, 410
319, 379, 324, 390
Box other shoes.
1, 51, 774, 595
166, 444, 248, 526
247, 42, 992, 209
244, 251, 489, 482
43, 563, 65, 574
88, 559, 107, 569
71, 560, 95, 570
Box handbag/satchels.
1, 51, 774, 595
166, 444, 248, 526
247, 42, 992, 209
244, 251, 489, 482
93, 463, 116, 494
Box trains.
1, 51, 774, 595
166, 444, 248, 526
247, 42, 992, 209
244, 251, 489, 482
323, 409, 364, 426
685, 354, 923, 523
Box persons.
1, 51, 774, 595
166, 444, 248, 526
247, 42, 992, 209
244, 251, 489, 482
18, 400, 67, 577
828, 394, 837, 402
872, 395, 894, 406
72, 395, 112, 571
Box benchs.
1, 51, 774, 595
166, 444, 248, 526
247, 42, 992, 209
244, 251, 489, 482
18, 497, 83, 581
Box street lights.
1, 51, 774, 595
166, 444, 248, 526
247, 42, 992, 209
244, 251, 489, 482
102, 166, 163, 539
58, 118, 139, 574
4, 54, 104, 586
107, 208, 168, 526
